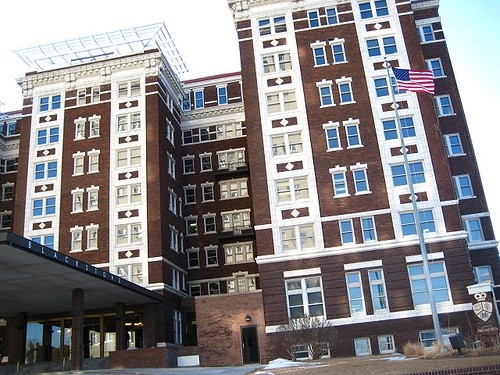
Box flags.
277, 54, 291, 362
391, 66, 435, 94
